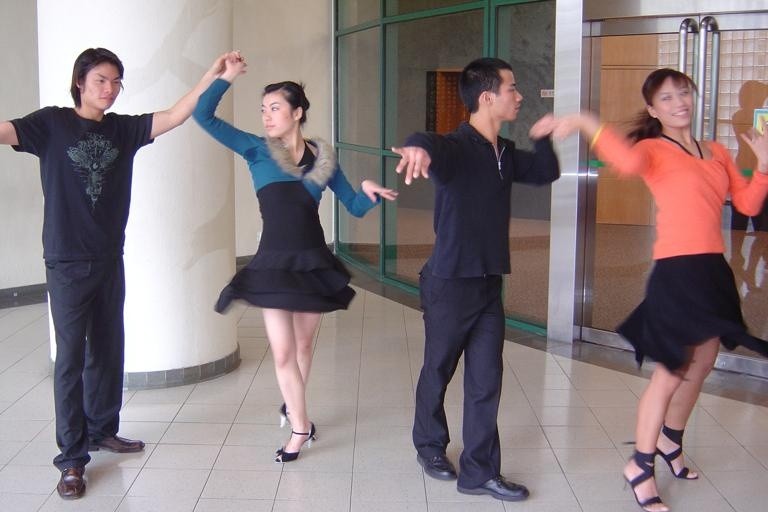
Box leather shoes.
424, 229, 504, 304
455, 465, 531, 501
86, 428, 145, 454
56, 462, 88, 500
415, 448, 458, 484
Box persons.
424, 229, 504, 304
0, 49, 242, 500
551, 68, 768, 511
731, 82, 768, 295
194, 55, 398, 464
391, 58, 560, 503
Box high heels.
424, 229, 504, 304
273, 421, 316, 465
620, 445, 671, 512
278, 401, 320, 443
651, 422, 700, 481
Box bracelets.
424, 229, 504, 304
590, 124, 605, 150
